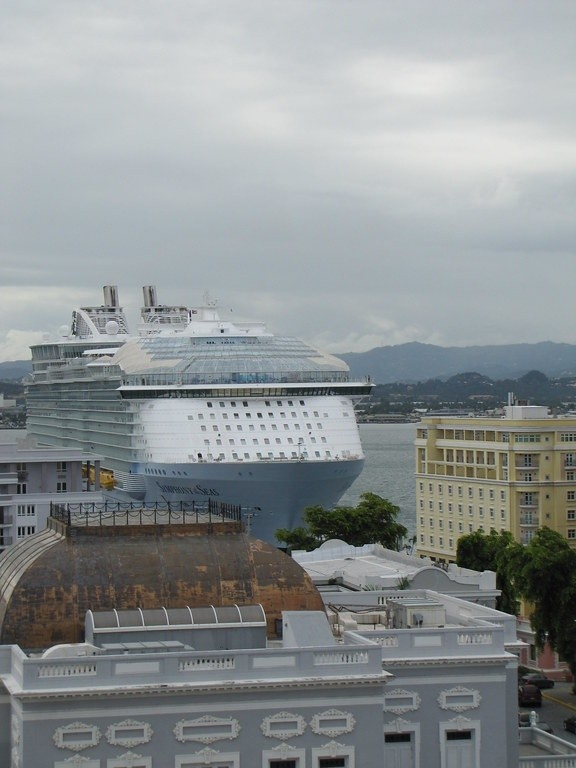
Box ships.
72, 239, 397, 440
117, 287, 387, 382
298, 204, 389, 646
24, 285, 364, 549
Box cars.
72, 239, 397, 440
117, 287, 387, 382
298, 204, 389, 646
520, 673, 555, 689
518, 684, 542, 708
518, 712, 553, 735
563, 716, 576, 736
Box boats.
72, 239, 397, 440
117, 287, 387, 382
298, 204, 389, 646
82, 465, 87, 478
90, 468, 113, 489
